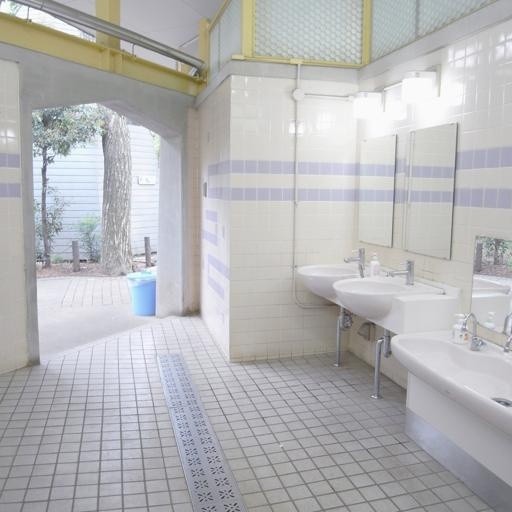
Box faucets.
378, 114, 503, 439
503, 311, 512, 332
463, 310, 483, 351
344, 248, 366, 278
386, 258, 415, 286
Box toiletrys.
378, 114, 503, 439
453, 313, 469, 344
370, 252, 381, 276
485, 311, 496, 330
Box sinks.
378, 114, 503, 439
296, 263, 361, 301
333, 277, 445, 320
390, 332, 512, 434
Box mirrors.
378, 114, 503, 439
358, 122, 458, 261
